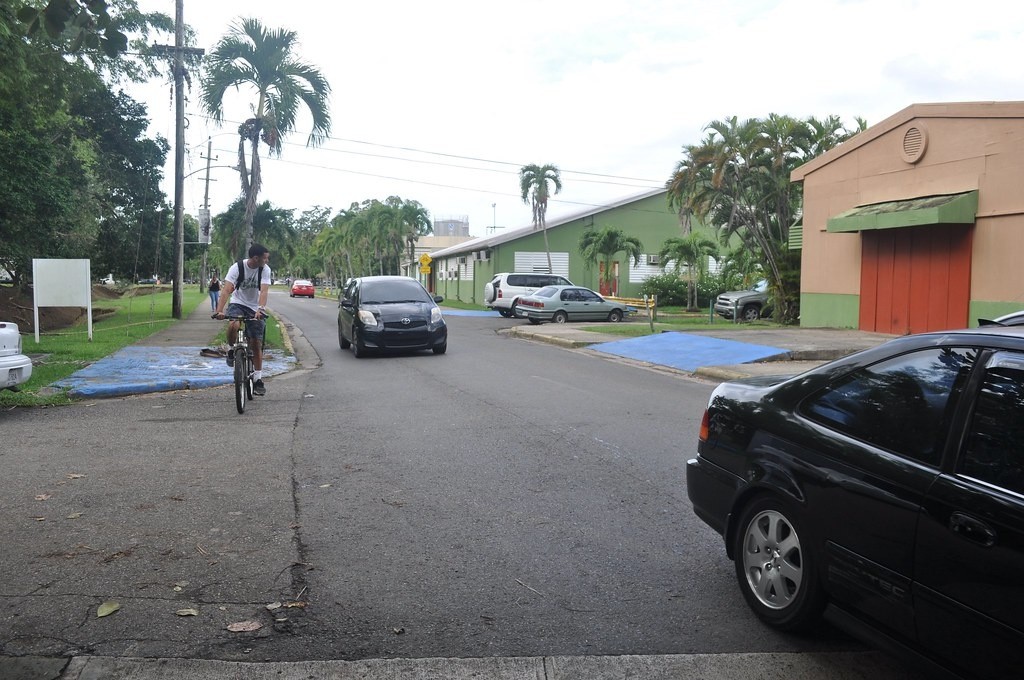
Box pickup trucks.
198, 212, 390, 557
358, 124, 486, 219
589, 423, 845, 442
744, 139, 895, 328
99, 278, 121, 285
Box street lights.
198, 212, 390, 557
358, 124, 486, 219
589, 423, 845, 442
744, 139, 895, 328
171, 165, 254, 319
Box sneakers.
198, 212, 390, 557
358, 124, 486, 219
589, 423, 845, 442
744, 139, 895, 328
253, 379, 266, 396
226, 349, 235, 367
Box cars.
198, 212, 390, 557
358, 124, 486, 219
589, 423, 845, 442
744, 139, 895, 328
271, 277, 331, 286
337, 275, 448, 359
0, 321, 32, 393
712, 278, 800, 325
683, 310, 1024, 680
289, 279, 315, 298
515, 285, 629, 325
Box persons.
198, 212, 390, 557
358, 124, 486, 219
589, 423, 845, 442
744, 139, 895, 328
212, 244, 271, 395
207, 275, 221, 311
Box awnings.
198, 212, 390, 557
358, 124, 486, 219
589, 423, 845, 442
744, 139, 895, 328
826, 192, 977, 234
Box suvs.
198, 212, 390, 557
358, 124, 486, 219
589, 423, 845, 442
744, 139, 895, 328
484, 271, 584, 319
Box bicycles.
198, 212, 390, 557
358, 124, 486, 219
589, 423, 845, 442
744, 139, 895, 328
209, 309, 270, 415
